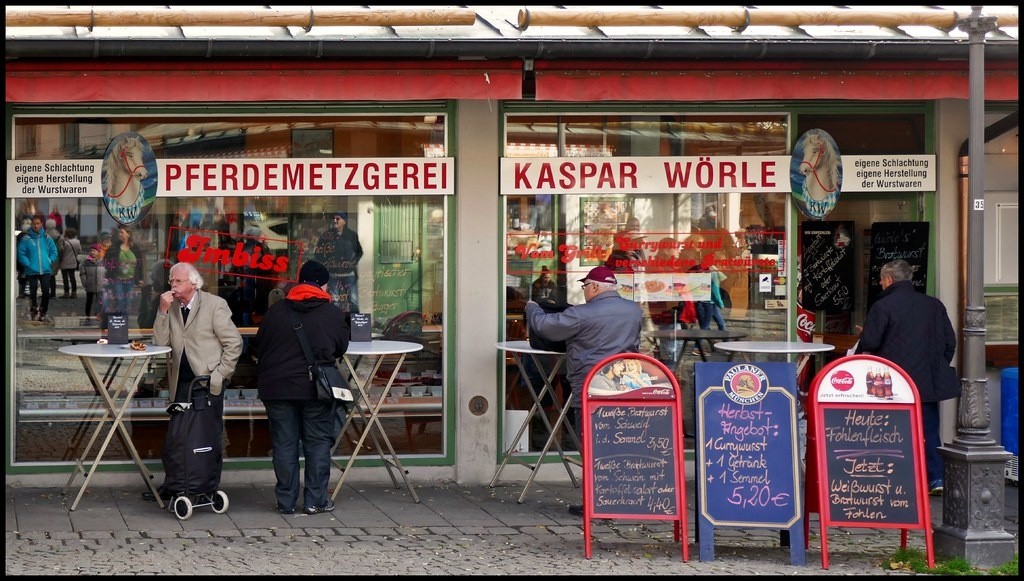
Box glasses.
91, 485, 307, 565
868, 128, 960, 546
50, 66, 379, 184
581, 283, 598, 289
168, 279, 189, 285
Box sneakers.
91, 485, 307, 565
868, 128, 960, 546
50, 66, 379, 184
276, 500, 294, 514
302, 499, 334, 514
929, 479, 943, 495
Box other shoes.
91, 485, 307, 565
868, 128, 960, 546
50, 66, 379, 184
49, 293, 55, 298
60, 293, 69, 299
691, 348, 712, 356
40, 315, 49, 321
32, 313, 39, 321
70, 292, 76, 298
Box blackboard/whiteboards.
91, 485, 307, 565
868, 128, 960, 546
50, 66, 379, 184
694, 362, 804, 529
580, 352, 687, 519
868, 222, 929, 310
801, 221, 855, 312
803, 354, 932, 530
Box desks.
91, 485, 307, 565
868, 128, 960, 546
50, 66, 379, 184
327, 340, 422, 507
713, 340, 835, 377
489, 335, 582, 506
58, 341, 173, 512
651, 324, 747, 376
50, 335, 158, 458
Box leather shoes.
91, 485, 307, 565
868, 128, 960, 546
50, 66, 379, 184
569, 504, 584, 516
143, 486, 172, 501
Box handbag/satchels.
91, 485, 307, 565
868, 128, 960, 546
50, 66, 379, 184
76, 260, 79, 270
528, 301, 574, 353
310, 364, 354, 403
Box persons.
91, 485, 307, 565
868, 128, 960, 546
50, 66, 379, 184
532, 265, 556, 306
594, 204, 728, 383
241, 227, 278, 304
855, 260, 955, 495
315, 212, 363, 313
142, 262, 243, 501
16, 202, 230, 325
254, 262, 350, 512
525, 267, 644, 514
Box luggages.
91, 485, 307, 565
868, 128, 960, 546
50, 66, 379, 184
162, 374, 230, 521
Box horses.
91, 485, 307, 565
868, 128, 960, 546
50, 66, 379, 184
102, 134, 148, 222
799, 132, 839, 216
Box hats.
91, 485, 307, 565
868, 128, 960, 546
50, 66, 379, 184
578, 266, 617, 284
624, 230, 647, 240
333, 210, 346, 220
299, 260, 329, 287
246, 227, 262, 237
90, 244, 101, 252
